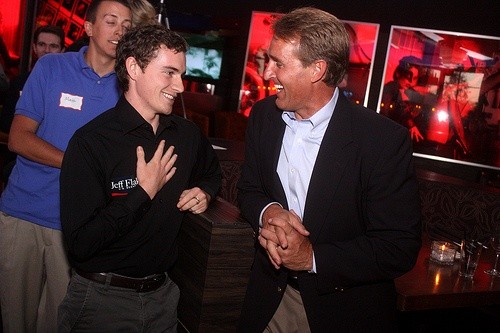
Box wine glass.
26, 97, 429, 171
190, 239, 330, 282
483, 236, 500, 277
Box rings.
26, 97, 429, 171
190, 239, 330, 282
193, 197, 201, 204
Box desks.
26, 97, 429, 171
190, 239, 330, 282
392, 241, 500, 333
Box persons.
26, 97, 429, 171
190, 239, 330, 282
380, 47, 500, 161
0, 25, 67, 191
0, 0, 132, 333
126, 0, 160, 30
236, 8, 423, 333
54, 24, 223, 333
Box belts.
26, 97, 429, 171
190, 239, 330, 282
71, 260, 166, 292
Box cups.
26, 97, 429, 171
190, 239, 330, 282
457, 240, 481, 278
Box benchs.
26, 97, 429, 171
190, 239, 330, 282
218, 160, 500, 242
